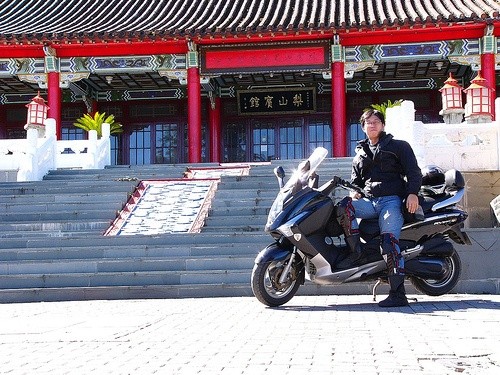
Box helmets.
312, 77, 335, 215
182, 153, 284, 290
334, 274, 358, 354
421, 164, 446, 195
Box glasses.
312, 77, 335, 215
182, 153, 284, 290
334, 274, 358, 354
364, 120, 382, 125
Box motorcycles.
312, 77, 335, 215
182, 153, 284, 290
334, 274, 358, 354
251, 147, 473, 308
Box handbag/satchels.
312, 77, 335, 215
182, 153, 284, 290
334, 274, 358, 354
401, 203, 425, 222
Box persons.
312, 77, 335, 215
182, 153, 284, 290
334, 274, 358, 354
335, 110, 423, 307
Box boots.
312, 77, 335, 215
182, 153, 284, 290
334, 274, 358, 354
378, 274, 408, 307
334, 234, 368, 270
